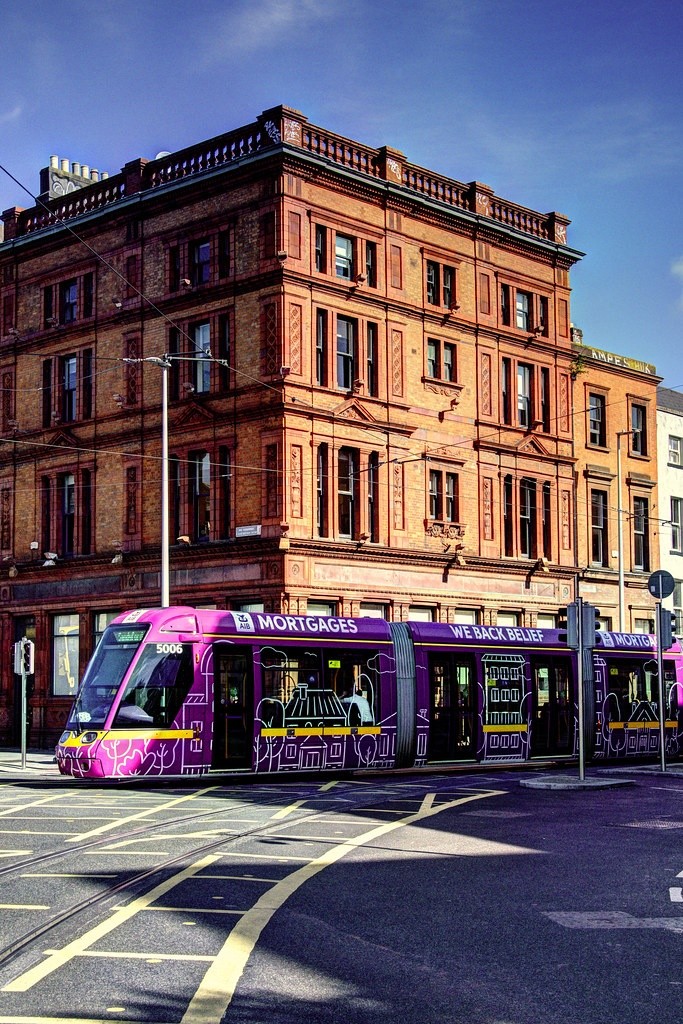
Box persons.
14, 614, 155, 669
143, 689, 165, 724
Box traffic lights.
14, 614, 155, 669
22, 639, 34, 676
557, 602, 579, 649
661, 607, 677, 651
11, 640, 23, 676
582, 600, 601, 650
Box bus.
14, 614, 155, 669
52, 602, 682, 786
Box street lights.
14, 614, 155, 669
616, 425, 642, 633
